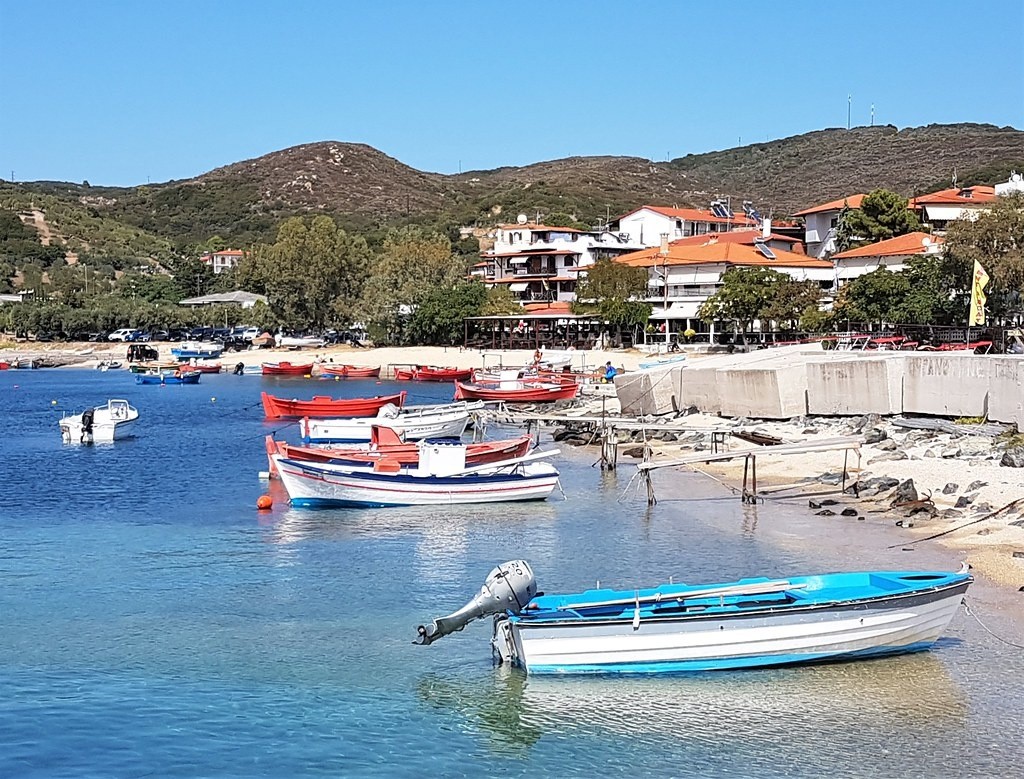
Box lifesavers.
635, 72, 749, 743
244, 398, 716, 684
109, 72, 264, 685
234, 362, 244, 373
175, 371, 181, 377
534, 354, 541, 362
342, 367, 348, 374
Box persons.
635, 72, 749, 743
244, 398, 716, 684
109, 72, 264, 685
313, 351, 335, 364
531, 348, 543, 370
601, 361, 617, 384
518, 320, 524, 330
151, 327, 156, 340
644, 321, 680, 352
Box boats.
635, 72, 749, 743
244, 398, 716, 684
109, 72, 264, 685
0, 358, 123, 371
261, 359, 316, 375
263, 423, 533, 480
393, 365, 475, 382
275, 438, 562, 507
128, 361, 222, 374
134, 370, 202, 385
59, 398, 140, 443
282, 338, 324, 346
171, 345, 221, 362
261, 390, 410, 419
409, 560, 975, 675
252, 337, 275, 346
453, 379, 578, 404
298, 400, 485, 442
317, 363, 381, 377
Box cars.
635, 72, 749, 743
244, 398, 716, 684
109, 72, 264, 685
322, 331, 360, 344
38, 324, 261, 353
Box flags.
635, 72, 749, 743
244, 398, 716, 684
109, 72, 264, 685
969, 259, 989, 327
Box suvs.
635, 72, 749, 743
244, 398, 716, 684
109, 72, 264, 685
126, 344, 159, 362
